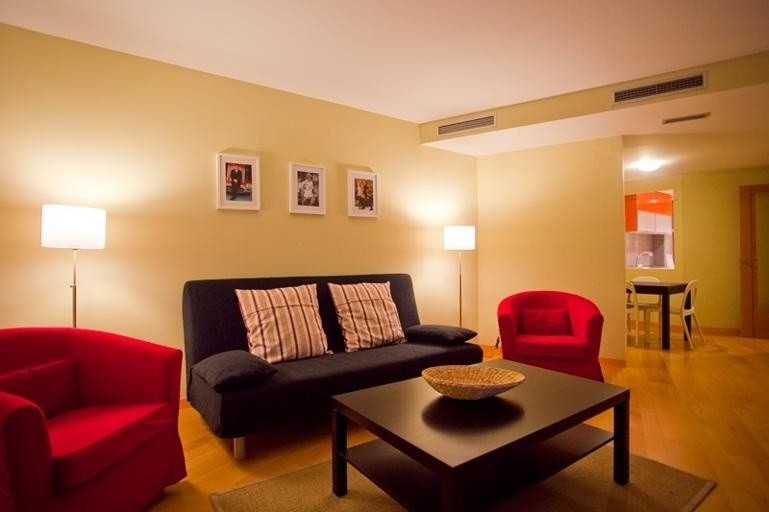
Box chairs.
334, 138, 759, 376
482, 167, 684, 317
629, 272, 664, 344
659, 279, 708, 352
1, 323, 192, 511
624, 280, 648, 352
494, 289, 607, 383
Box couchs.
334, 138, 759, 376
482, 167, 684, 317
180, 272, 485, 463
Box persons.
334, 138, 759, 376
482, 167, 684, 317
230, 164, 242, 200
300, 173, 316, 206
358, 180, 372, 211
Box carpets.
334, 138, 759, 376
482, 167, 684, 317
207, 432, 719, 511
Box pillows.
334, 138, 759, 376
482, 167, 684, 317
230, 277, 333, 365
326, 273, 415, 352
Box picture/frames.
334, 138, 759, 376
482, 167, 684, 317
212, 150, 264, 213
343, 164, 378, 220
287, 160, 329, 218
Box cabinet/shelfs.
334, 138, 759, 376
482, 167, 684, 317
624, 191, 674, 236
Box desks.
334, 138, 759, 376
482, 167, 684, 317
626, 281, 695, 350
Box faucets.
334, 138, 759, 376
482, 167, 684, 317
635, 251, 653, 267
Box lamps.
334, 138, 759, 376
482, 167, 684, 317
36, 198, 111, 330
438, 221, 480, 329
